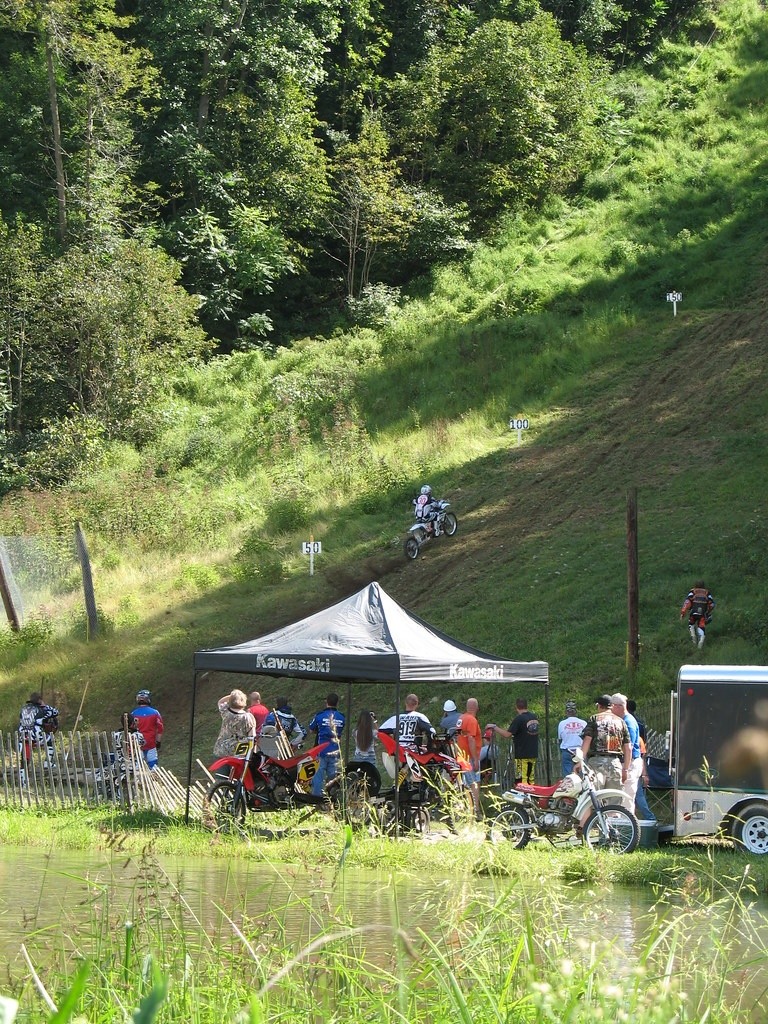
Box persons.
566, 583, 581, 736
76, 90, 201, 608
681, 580, 715, 648
440, 697, 499, 821
114, 689, 163, 770
486, 697, 539, 784
415, 485, 444, 536
18, 692, 59, 788
558, 692, 656, 840
213, 690, 307, 781
352, 710, 379, 768
309, 693, 346, 796
379, 694, 436, 755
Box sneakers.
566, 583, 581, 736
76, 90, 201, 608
43, 761, 56, 768
22, 783, 31, 789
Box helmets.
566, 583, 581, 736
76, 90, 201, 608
136, 689, 151, 704
444, 700, 456, 712
421, 485, 432, 494
43, 718, 59, 734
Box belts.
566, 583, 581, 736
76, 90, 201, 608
589, 754, 619, 758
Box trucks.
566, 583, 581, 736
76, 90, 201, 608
666, 664, 768, 859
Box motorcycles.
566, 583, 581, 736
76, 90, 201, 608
202, 725, 381, 830
486, 746, 642, 857
344, 719, 477, 836
404, 500, 458, 559
91, 717, 143, 804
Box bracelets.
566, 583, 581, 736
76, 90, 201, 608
492, 724, 496, 729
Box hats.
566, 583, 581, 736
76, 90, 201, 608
596, 694, 612, 706
610, 693, 628, 705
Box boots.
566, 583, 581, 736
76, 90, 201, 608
433, 521, 444, 536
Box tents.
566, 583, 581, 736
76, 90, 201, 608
185, 582, 551, 842
